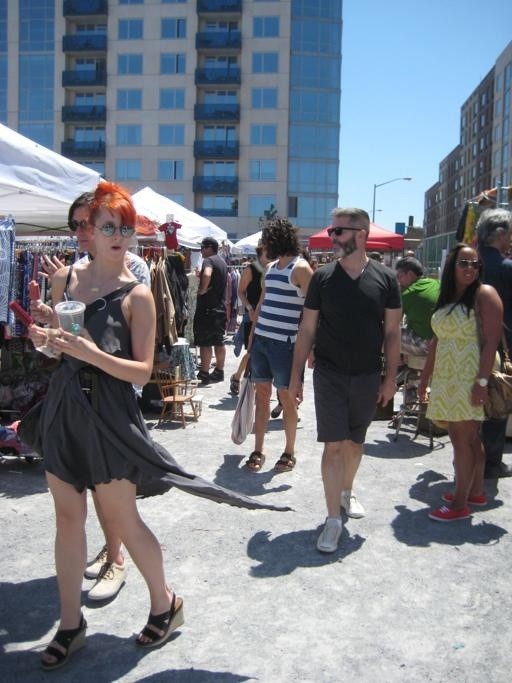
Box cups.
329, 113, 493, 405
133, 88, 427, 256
55, 300, 86, 333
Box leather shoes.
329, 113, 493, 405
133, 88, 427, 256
484, 463, 512, 478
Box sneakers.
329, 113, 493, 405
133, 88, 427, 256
271, 403, 282, 418
441, 490, 487, 506
339, 489, 364, 519
230, 373, 240, 395
428, 504, 469, 522
316, 518, 342, 553
197, 370, 209, 385
87, 550, 130, 600
209, 367, 224, 380
84, 543, 109, 579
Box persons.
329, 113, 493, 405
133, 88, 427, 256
245, 217, 315, 473
416, 243, 504, 522
29, 191, 152, 601
192, 235, 228, 385
27, 182, 190, 671
271, 249, 312, 424
381, 255, 442, 385
475, 208, 512, 479
229, 238, 269, 395
288, 206, 403, 554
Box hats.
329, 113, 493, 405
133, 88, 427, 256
197, 236, 218, 248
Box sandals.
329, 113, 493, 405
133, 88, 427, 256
274, 452, 296, 472
246, 451, 265, 471
135, 591, 185, 647
39, 612, 87, 671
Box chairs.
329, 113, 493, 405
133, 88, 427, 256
151, 361, 200, 426
174, 364, 201, 419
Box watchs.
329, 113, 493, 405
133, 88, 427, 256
474, 377, 489, 388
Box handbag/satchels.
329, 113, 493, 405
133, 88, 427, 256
231, 358, 254, 445
474, 283, 512, 421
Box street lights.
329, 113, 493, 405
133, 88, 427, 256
373, 177, 412, 223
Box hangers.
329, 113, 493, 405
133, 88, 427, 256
131, 243, 168, 270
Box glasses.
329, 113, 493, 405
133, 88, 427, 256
93, 221, 136, 239
68, 219, 86, 231
327, 227, 361, 237
456, 260, 482, 270
